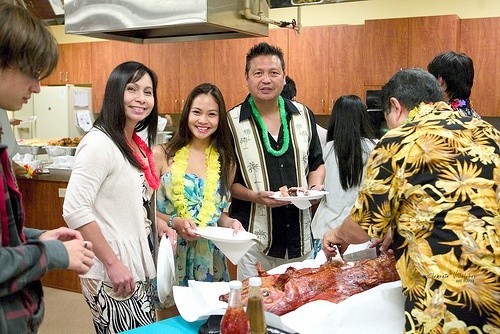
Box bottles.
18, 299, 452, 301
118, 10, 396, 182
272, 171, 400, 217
220, 280, 251, 334
246, 277, 268, 334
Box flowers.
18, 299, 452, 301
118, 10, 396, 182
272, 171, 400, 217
24, 164, 37, 179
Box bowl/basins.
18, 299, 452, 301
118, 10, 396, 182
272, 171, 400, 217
44, 145, 76, 156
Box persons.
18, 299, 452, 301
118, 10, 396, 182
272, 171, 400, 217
280, 75, 297, 102
312, 96, 379, 257
0, 1, 95, 334
427, 52, 483, 121
63, 61, 177, 334
323, 68, 500, 334
151, 83, 247, 322
226, 42, 325, 270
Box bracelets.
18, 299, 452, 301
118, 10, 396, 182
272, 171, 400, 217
310, 184, 316, 190
169, 213, 177, 228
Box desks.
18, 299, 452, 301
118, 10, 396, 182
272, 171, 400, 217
16, 165, 83, 294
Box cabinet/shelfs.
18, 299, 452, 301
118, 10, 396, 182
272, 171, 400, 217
38, 15, 500, 119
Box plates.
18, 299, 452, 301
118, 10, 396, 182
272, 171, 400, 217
191, 227, 257, 242
268, 190, 329, 201
156, 234, 175, 303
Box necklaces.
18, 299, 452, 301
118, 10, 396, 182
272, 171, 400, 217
117, 128, 160, 190
249, 96, 289, 156
170, 140, 220, 227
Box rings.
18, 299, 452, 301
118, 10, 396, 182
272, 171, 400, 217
184, 226, 190, 230
173, 239, 177, 245
83, 240, 88, 249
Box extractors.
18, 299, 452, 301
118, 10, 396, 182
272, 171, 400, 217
63, 0, 270, 45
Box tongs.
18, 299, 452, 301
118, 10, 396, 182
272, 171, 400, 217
327, 242, 345, 264
